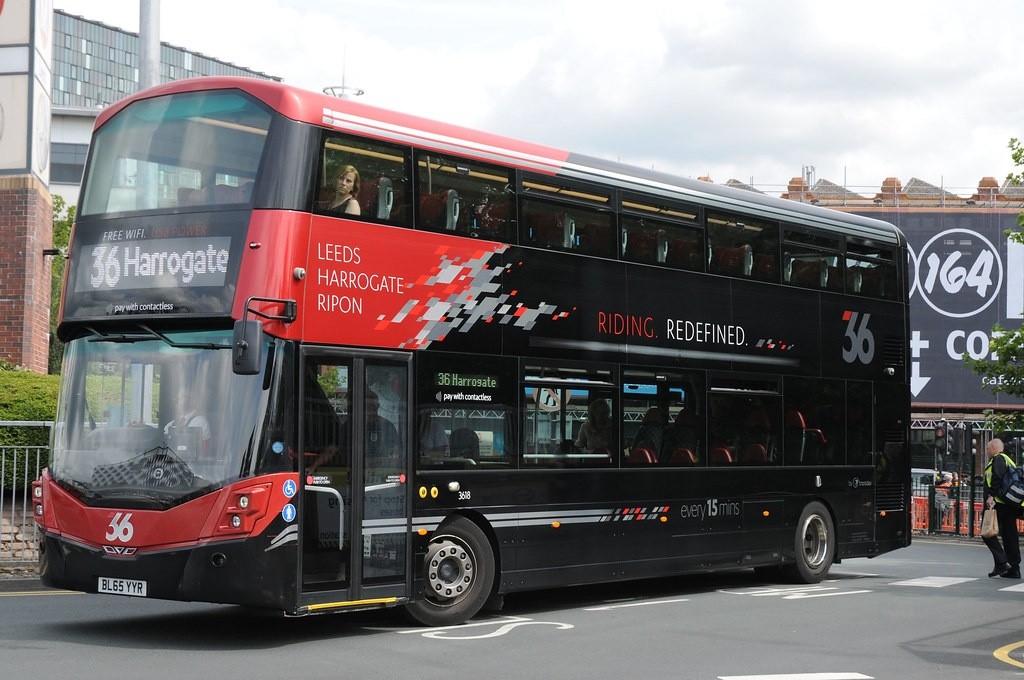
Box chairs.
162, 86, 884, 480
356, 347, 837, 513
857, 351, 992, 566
593, 410, 833, 466
178, 176, 878, 293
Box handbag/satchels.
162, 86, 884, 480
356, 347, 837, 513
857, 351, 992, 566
996, 462, 1024, 506
981, 506, 999, 539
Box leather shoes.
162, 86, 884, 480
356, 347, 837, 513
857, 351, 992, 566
999, 564, 1021, 578
988, 562, 1012, 577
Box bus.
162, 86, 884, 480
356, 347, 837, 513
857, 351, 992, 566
32, 76, 913, 627
911, 468, 952, 497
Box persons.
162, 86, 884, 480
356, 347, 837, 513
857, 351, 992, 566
708, 392, 741, 464
418, 399, 448, 464
575, 397, 612, 455
935, 472, 983, 525
981, 438, 1024, 579
305, 388, 403, 481
164, 376, 257, 458
325, 166, 362, 217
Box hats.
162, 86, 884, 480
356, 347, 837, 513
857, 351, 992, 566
943, 474, 953, 480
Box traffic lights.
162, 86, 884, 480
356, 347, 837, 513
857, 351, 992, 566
936, 425, 945, 449
1017, 437, 1024, 466
1007, 436, 1020, 465
941, 421, 954, 456
965, 422, 979, 457
